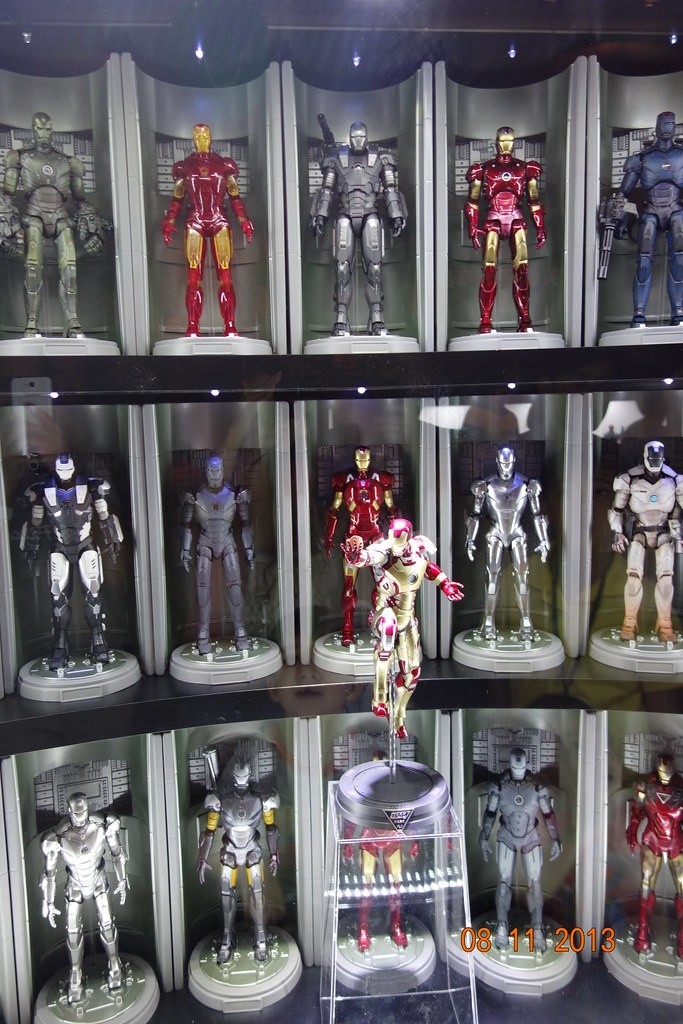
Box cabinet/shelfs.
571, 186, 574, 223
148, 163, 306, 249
1, 6, 683, 1024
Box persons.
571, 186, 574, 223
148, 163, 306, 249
162, 123, 254, 337
310, 122, 408, 336
0, 112, 113, 339
615, 112, 683, 328
24, 454, 124, 670
479, 748, 562, 952
39, 792, 131, 1002
343, 750, 420, 951
464, 127, 547, 333
607, 441, 683, 643
180, 456, 255, 655
341, 519, 464, 739
325, 446, 403, 646
464, 447, 550, 642
626, 752, 683, 958
197, 764, 282, 964
